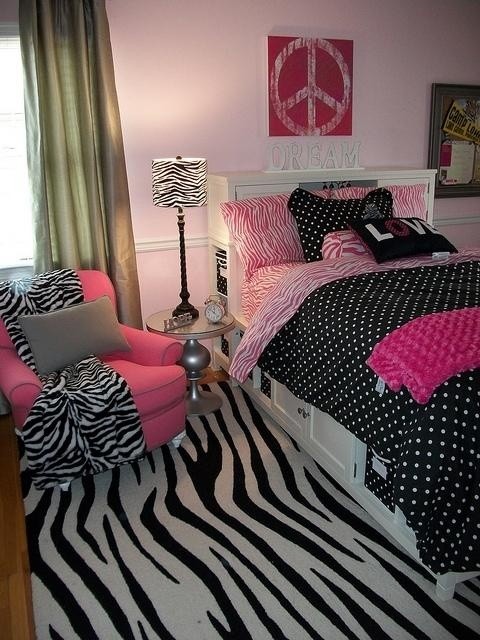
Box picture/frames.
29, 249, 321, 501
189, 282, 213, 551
424, 81, 480, 201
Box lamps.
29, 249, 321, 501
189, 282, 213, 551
151, 155, 209, 322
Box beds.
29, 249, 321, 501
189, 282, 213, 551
205, 166, 480, 604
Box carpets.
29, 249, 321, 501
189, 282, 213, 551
11, 378, 480, 640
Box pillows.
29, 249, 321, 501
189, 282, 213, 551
329, 181, 429, 221
218, 186, 334, 279
321, 229, 377, 263
343, 213, 460, 266
288, 183, 392, 263
16, 293, 134, 376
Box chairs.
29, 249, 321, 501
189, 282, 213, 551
0, 267, 191, 493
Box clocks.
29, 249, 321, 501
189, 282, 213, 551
204, 294, 228, 326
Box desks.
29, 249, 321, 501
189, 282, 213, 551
142, 300, 236, 420
